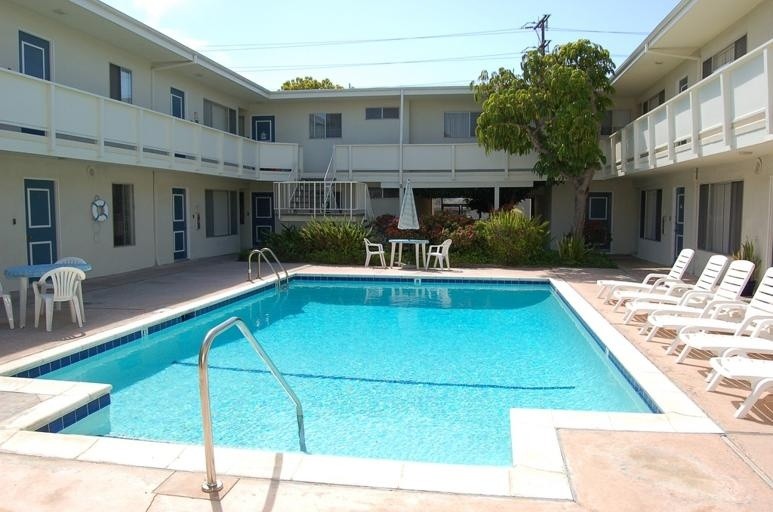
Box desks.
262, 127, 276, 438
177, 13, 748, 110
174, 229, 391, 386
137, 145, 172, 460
389, 237, 429, 270
5, 265, 93, 328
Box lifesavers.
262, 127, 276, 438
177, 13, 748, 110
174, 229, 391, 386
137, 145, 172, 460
92, 200, 108, 221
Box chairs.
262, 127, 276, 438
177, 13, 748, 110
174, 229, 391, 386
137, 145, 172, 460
596, 248, 773, 419
364, 237, 384, 269
426, 238, 454, 270
0, 256, 85, 332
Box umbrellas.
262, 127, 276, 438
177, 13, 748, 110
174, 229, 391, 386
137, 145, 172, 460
398, 179, 420, 264
